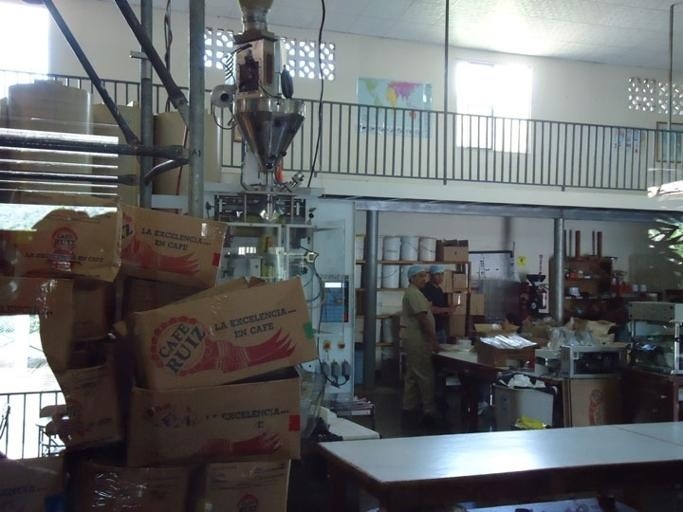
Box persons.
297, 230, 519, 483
401, 264, 446, 420
422, 264, 454, 345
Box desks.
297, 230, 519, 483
438, 350, 567, 430
319, 420, 683, 512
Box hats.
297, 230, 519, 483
409, 266, 425, 279
430, 265, 444, 274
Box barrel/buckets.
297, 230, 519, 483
381, 233, 447, 290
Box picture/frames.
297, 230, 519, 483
655, 121, 683, 166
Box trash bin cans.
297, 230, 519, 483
493, 384, 555, 430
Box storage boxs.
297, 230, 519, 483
0, 201, 126, 511
126, 365, 304, 462
116, 276, 316, 389
66, 455, 191, 511
194, 458, 292, 512
436, 237, 485, 335
113, 203, 228, 281
121, 278, 191, 322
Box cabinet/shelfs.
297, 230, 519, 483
549, 256, 613, 325
353, 260, 472, 349
